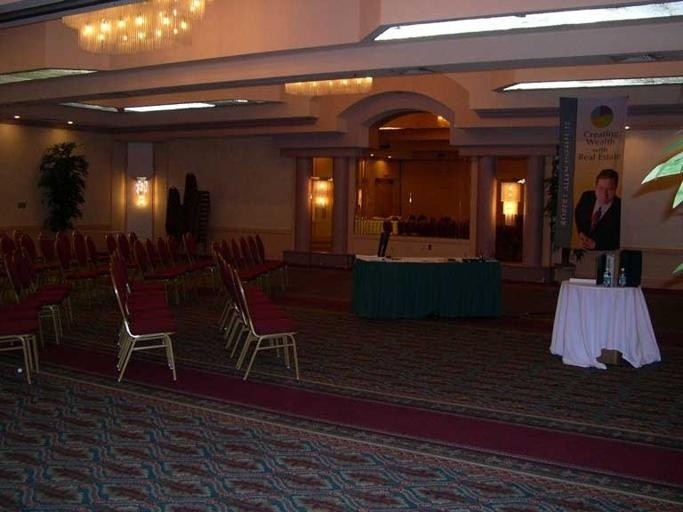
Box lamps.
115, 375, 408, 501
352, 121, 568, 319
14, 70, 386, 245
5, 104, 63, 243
501, 181, 520, 226
63, 1, 205, 57
286, 77, 374, 97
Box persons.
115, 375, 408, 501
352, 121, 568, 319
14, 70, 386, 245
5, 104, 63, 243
572, 168, 620, 250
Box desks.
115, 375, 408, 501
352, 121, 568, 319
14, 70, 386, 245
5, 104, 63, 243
549, 281, 662, 370
352, 254, 503, 321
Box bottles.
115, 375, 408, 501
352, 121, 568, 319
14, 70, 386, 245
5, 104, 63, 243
618, 267, 626, 287
603, 267, 611, 287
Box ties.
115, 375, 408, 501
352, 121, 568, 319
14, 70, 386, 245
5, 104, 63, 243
591, 206, 601, 233
